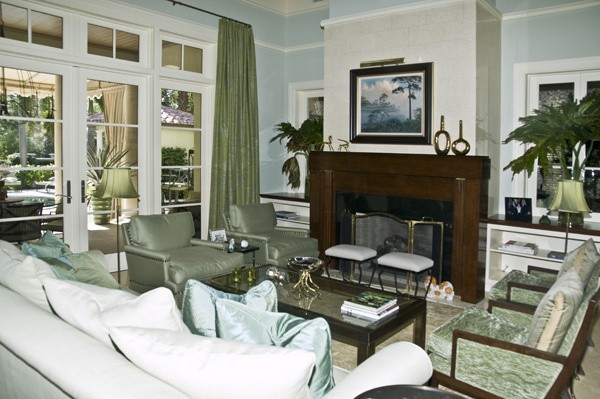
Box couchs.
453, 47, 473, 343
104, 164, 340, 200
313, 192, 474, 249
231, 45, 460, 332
223, 202, 320, 267
1, 240, 335, 397
122, 211, 246, 310
427, 289, 600, 399
488, 238, 600, 309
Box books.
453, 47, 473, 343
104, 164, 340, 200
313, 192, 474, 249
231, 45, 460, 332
547, 250, 567, 260
498, 240, 539, 255
340, 290, 400, 320
275, 211, 300, 220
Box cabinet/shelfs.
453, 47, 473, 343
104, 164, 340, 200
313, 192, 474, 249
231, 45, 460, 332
261, 196, 311, 233
486, 230, 600, 290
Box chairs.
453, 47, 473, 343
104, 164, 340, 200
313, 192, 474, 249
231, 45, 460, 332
41, 198, 63, 238
34, 177, 55, 193
1, 203, 42, 243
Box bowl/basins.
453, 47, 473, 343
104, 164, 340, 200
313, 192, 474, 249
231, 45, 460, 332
289, 257, 322, 270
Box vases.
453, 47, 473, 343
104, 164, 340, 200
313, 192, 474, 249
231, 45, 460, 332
2, 180, 8, 199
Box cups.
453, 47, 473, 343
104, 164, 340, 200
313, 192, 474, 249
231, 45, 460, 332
232, 268, 244, 283
245, 268, 257, 284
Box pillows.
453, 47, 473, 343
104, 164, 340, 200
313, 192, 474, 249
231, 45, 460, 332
183, 278, 278, 339
557, 238, 600, 290
0, 242, 54, 314
103, 286, 191, 335
521, 268, 584, 355
43, 277, 137, 351
110, 326, 317, 399
215, 297, 336, 398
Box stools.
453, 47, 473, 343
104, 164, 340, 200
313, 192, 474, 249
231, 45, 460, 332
324, 243, 380, 289
373, 252, 434, 298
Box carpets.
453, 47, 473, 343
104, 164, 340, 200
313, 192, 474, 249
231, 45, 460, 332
329, 292, 476, 374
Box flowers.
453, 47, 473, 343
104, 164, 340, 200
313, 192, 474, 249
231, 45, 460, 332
0, 165, 10, 179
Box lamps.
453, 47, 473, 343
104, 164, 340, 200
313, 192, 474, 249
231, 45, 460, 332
93, 167, 140, 285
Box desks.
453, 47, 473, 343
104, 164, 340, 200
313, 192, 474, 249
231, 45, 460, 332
162, 185, 185, 204
1, 191, 61, 208
226, 239, 260, 269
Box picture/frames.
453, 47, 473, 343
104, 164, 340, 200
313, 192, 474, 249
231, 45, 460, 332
505, 196, 532, 223
348, 61, 434, 143
208, 228, 227, 243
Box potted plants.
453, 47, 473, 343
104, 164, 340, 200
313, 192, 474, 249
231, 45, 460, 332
86, 145, 129, 225
271, 118, 323, 200
500, 91, 600, 225
